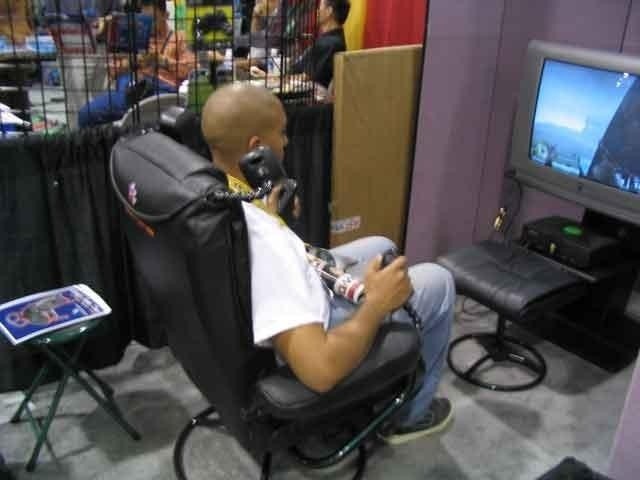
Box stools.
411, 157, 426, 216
436, 237, 590, 394
8, 308, 142, 470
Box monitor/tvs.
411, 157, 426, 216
506, 37, 640, 226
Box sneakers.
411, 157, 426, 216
376, 398, 454, 445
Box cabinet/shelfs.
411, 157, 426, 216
515, 241, 640, 375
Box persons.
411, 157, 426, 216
76, 0, 199, 130
222, 0, 279, 66
226, 0, 352, 97
198, 82, 456, 445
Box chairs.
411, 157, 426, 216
110, 132, 421, 478
